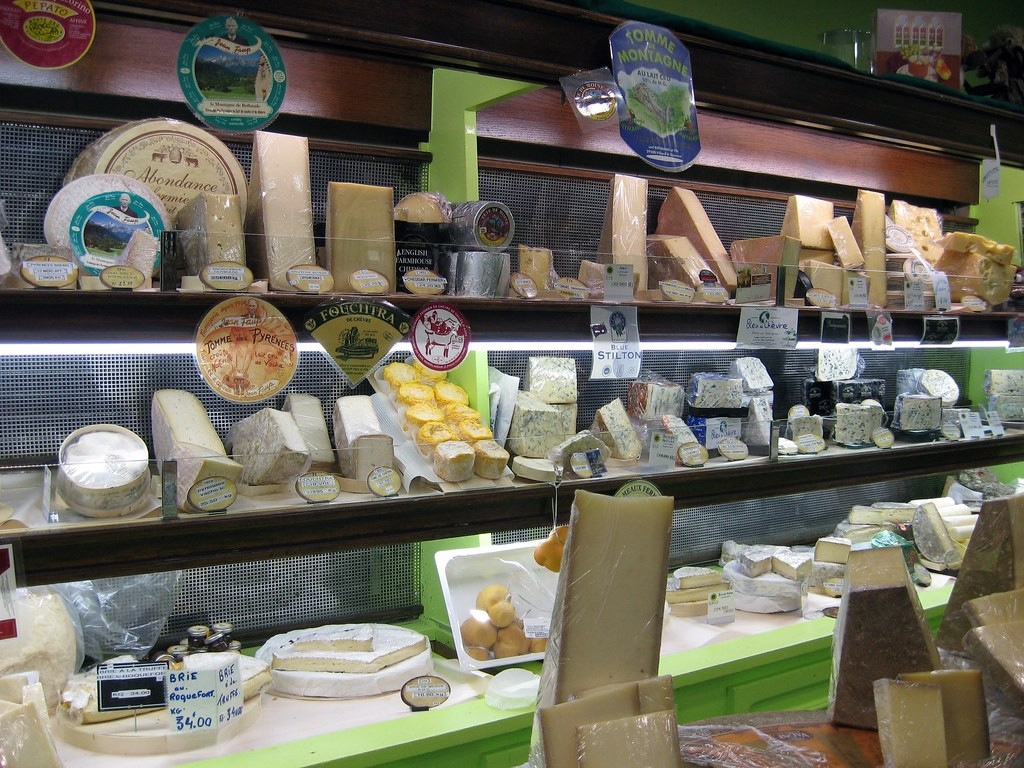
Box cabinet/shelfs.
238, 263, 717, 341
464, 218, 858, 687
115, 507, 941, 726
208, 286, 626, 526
0, 0, 1024, 768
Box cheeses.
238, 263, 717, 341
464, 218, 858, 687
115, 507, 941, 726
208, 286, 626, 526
0, 118, 1024, 768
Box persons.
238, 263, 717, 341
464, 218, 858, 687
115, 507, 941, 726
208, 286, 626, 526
220, 17, 250, 47
113, 193, 139, 218
